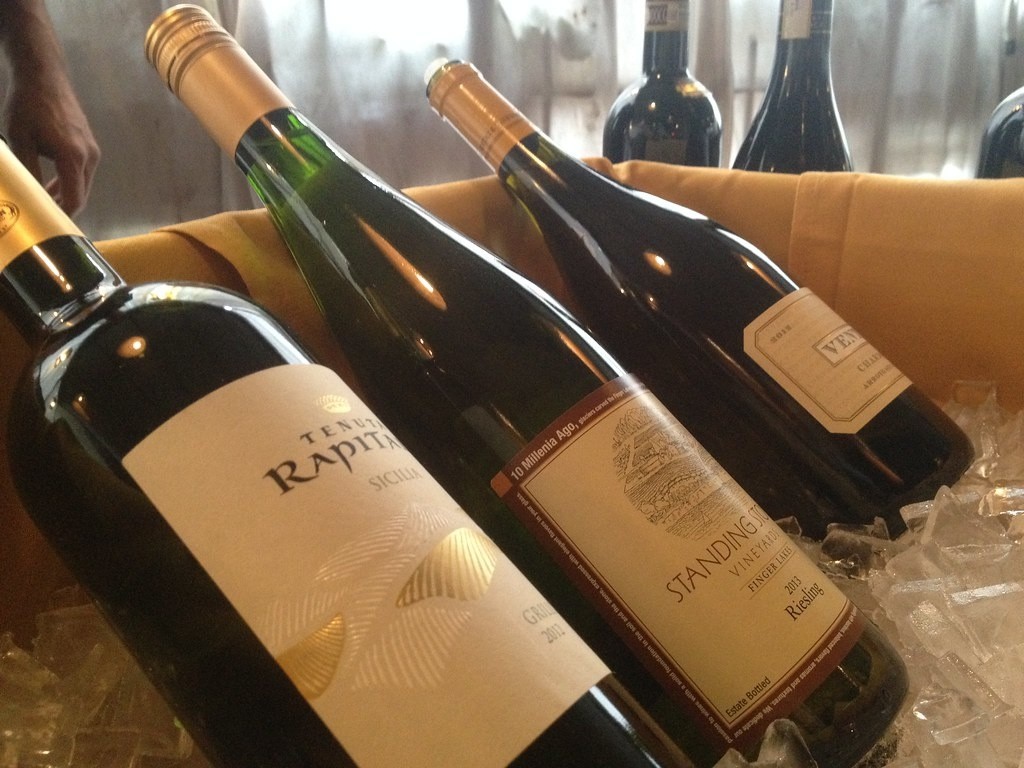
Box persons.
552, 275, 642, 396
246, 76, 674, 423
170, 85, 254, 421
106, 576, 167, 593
0, 0, 102, 222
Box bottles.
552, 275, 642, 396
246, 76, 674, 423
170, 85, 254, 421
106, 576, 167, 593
733, 0, 855, 174
425, 56, 976, 541
603, 0, 724, 167
976, 86, 1024, 179
0, 136, 681, 768
142, 4, 911, 768
